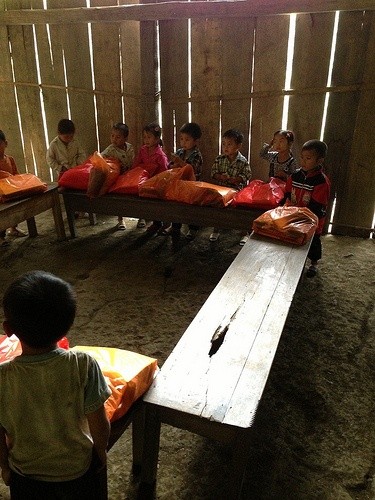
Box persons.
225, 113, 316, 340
208, 129, 253, 246
134, 122, 169, 236
262, 129, 297, 182
167, 123, 204, 240
291, 139, 331, 278
0, 271, 113, 500
46, 119, 90, 219
0, 129, 29, 247
100, 122, 146, 231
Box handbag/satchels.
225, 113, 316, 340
253, 205, 318, 246
58, 152, 92, 190
0, 171, 48, 202
176, 180, 239, 208
87, 151, 121, 194
230, 176, 287, 210
139, 164, 196, 199
70, 346, 160, 425
109, 166, 156, 195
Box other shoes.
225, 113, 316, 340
306, 261, 317, 276
185, 229, 199, 241
10, 227, 29, 237
210, 233, 220, 241
0, 229, 6, 240
240, 234, 249, 245
116, 223, 126, 229
162, 226, 180, 235
147, 223, 162, 234
137, 219, 146, 227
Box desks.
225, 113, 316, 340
131, 229, 316, 498
57, 188, 264, 239
0, 182, 67, 242
